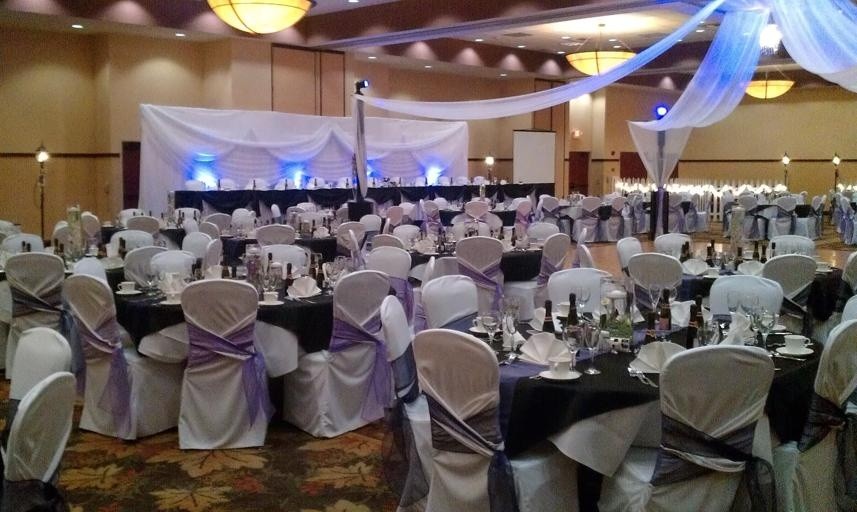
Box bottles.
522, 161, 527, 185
498, 226, 504, 240
309, 253, 317, 280
149, 209, 152, 216
182, 211, 185, 220
752, 241, 760, 261
52, 238, 60, 255
326, 218, 331, 233
464, 229, 479, 238
266, 252, 273, 273
528, 206, 536, 224
470, 176, 473, 184
685, 303, 699, 333
706, 246, 714, 267
760, 244, 767, 263
118, 237, 128, 259
312, 219, 317, 235
542, 298, 555, 333
314, 178, 317, 186
694, 292, 704, 329
217, 179, 221, 191
771, 242, 776, 257
567, 293, 579, 328
322, 216, 327, 227
511, 227, 517, 246
710, 238, 715, 257
59, 243, 66, 259
450, 177, 453, 185
178, 209, 183, 225
221, 266, 230, 278
425, 176, 427, 185
436, 227, 447, 252
679, 244, 687, 263
659, 288, 672, 330
345, 178, 349, 188
685, 241, 690, 259
252, 180, 256, 190
193, 210, 197, 221
316, 257, 324, 289
284, 262, 293, 296
26, 243, 31, 251
734, 245, 744, 270
232, 265, 237, 279
161, 212, 164, 220
192, 257, 203, 281
22, 239, 27, 251
285, 178, 288, 190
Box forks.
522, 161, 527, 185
629, 368, 659, 388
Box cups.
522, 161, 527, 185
784, 334, 811, 353
263, 292, 279, 302
548, 357, 572, 376
167, 293, 182, 305
473, 317, 482, 328
117, 281, 136, 293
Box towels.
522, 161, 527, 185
287, 277, 320, 298
670, 301, 703, 326
519, 334, 573, 367
500, 240, 512, 251
414, 239, 435, 253
500, 318, 525, 347
628, 341, 688, 374
205, 265, 224, 280
682, 259, 708, 274
730, 313, 749, 332
617, 306, 642, 322
160, 272, 187, 294
531, 309, 556, 328
714, 333, 744, 347
739, 262, 766, 275
314, 227, 329, 238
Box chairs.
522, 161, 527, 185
5, 233, 44, 255
128, 217, 160, 239
204, 213, 231, 235
720, 190, 738, 237
422, 275, 480, 337
337, 221, 365, 263
3, 371, 78, 512
393, 225, 420, 246
546, 267, 616, 325
579, 242, 627, 318
62, 274, 180, 442
232, 208, 254, 235
206, 239, 221, 281
174, 207, 200, 223
686, 194, 699, 233
297, 212, 323, 229
527, 223, 559, 245
762, 254, 816, 334
79, 212, 103, 245
798, 194, 827, 240
624, 192, 647, 237
654, 233, 692, 261
118, 208, 145, 225
255, 224, 295, 246
261, 245, 305, 279
182, 232, 212, 277
739, 195, 760, 240
697, 193, 711, 232
5, 253, 129, 405
73, 256, 108, 284
507, 233, 569, 321
454, 222, 489, 237
371, 234, 404, 250
200, 222, 219, 239
111, 229, 154, 262
151, 251, 192, 285
840, 197, 856, 244
709, 275, 782, 327
455, 236, 505, 319
413, 329, 580, 512
380, 294, 435, 460
504, 201, 530, 239
125, 247, 169, 284
777, 318, 857, 512
617, 237, 643, 275
628, 253, 683, 322
367, 246, 412, 322
595, 343, 776, 511
385, 208, 403, 237
572, 197, 598, 242
767, 234, 815, 260
667, 192, 685, 233
286, 207, 305, 222
836, 250, 856, 313
360, 214, 381, 249
464, 201, 488, 233
834, 193, 854, 233
767, 197, 795, 239
599, 197, 624, 242
424, 200, 441, 235
541, 197, 572, 242
284, 270, 392, 439
5, 326, 72, 438
178, 280, 272, 450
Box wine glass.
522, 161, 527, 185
584, 321, 602, 375
727, 291, 780, 357
322, 257, 358, 297
662, 284, 678, 310
144, 265, 158, 298
648, 283, 663, 313
712, 251, 736, 272
696, 321, 721, 347
261, 266, 283, 294
505, 310, 520, 357
568, 287, 591, 320
482, 312, 498, 354
563, 327, 584, 370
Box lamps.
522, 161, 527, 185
781, 150, 789, 187
737, 48, 794, 99
208, 0, 314, 35
36, 146, 52, 238
567, 23, 635, 76
832, 152, 840, 192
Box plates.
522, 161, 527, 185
539, 371, 582, 380
116, 290, 143, 295
776, 347, 814, 356
468, 327, 500, 333
158, 299, 184, 305
259, 301, 285, 306
